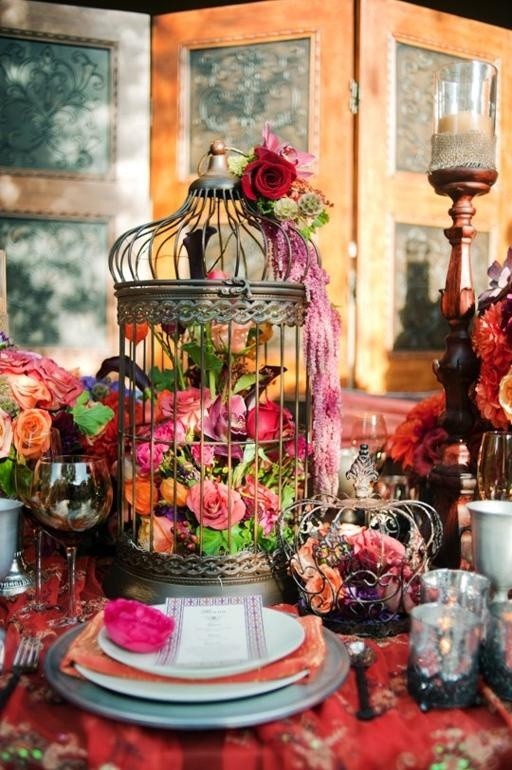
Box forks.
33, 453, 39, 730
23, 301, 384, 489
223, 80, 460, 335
1, 634, 42, 709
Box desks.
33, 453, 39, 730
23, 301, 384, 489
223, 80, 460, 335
0, 535, 512, 769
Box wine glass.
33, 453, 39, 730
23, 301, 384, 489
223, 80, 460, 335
28, 456, 114, 627
14, 426, 63, 613
465, 500, 512, 603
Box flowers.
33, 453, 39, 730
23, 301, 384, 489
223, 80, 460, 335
239, 151, 297, 206
294, 518, 426, 630
386, 398, 463, 480
466, 292, 512, 440
89, 594, 176, 645
0, 345, 310, 560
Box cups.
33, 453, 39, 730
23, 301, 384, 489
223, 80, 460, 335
484, 602, 512, 702
477, 430, 512, 500
350, 412, 387, 451
0, 498, 22, 584
419, 568, 492, 619
407, 602, 483, 710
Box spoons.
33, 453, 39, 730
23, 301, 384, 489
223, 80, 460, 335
347, 642, 377, 722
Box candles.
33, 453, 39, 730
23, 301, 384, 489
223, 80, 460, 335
436, 110, 493, 134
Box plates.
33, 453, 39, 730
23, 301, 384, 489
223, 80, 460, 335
42, 602, 350, 731
